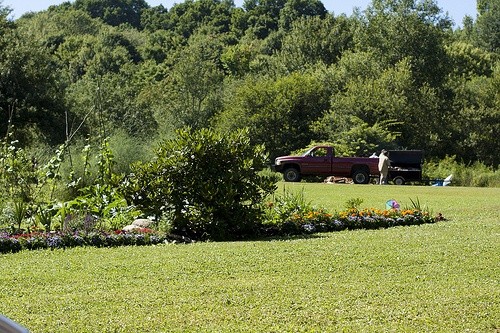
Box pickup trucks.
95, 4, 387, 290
274, 144, 380, 184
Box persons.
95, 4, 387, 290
379, 149, 384, 157
378, 151, 390, 185
369, 152, 379, 158
